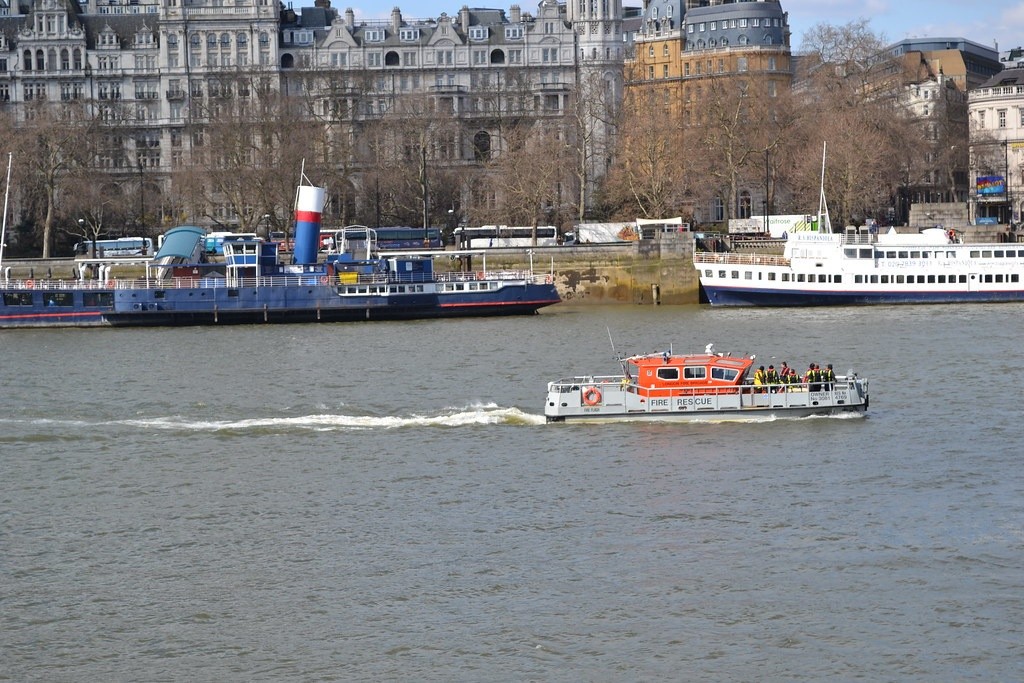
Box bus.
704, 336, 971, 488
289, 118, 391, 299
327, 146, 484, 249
157, 231, 265, 255
73, 236, 155, 258
269, 227, 339, 255
342, 226, 443, 252
453, 224, 558, 250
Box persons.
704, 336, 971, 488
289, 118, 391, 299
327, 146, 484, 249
824, 364, 837, 391
802, 363, 817, 392
753, 366, 767, 393
812, 364, 828, 392
779, 362, 791, 389
765, 365, 779, 393
779, 369, 802, 388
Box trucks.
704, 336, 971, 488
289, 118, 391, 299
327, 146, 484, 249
562, 220, 645, 247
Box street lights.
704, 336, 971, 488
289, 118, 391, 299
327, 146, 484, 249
1001, 137, 1009, 223
137, 162, 146, 240
764, 147, 770, 235
421, 148, 429, 241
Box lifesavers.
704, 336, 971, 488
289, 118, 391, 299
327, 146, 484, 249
106, 281, 117, 288
947, 230, 955, 237
320, 275, 330, 284
26, 280, 34, 290
711, 238, 723, 249
546, 275, 552, 283
583, 387, 602, 406
477, 270, 485, 281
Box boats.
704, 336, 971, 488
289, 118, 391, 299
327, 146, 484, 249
692, 139, 1024, 307
0, 156, 564, 330
545, 324, 869, 422
